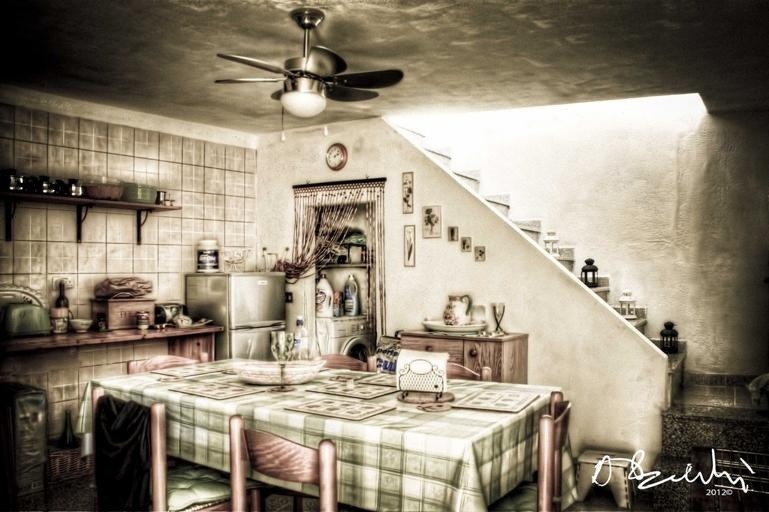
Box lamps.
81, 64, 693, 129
277, 73, 330, 142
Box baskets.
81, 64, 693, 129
45, 437, 96, 487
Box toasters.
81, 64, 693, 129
3, 303, 56, 338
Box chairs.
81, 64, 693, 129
126, 350, 211, 375
487, 390, 572, 512
227, 412, 343, 512
89, 383, 265, 512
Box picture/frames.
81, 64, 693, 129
421, 204, 442, 239
403, 224, 417, 268
401, 171, 414, 215
449, 224, 487, 261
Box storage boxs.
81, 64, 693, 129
89, 298, 156, 332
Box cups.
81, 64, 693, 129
157, 191, 166, 204
3, 168, 83, 199
50, 307, 69, 334
469, 305, 487, 325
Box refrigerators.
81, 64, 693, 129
184, 272, 289, 361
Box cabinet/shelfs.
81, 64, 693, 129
395, 326, 531, 386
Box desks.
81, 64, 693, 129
1, 323, 225, 366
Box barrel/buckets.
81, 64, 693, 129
343, 274, 361, 315
316, 271, 335, 319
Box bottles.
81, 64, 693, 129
56, 281, 68, 307
60, 409, 76, 449
195, 239, 221, 273
293, 315, 309, 361
97, 312, 107, 332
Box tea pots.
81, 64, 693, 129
444, 294, 475, 327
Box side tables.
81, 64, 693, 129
74, 357, 580, 511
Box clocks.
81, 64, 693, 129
325, 142, 348, 171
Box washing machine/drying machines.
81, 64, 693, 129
317, 317, 378, 368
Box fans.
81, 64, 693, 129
215, 3, 406, 102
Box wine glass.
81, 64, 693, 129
269, 331, 297, 393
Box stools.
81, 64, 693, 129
574, 447, 637, 511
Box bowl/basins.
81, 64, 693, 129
83, 183, 125, 200
230, 359, 328, 385
69, 319, 95, 334
423, 320, 488, 333
121, 181, 159, 204
